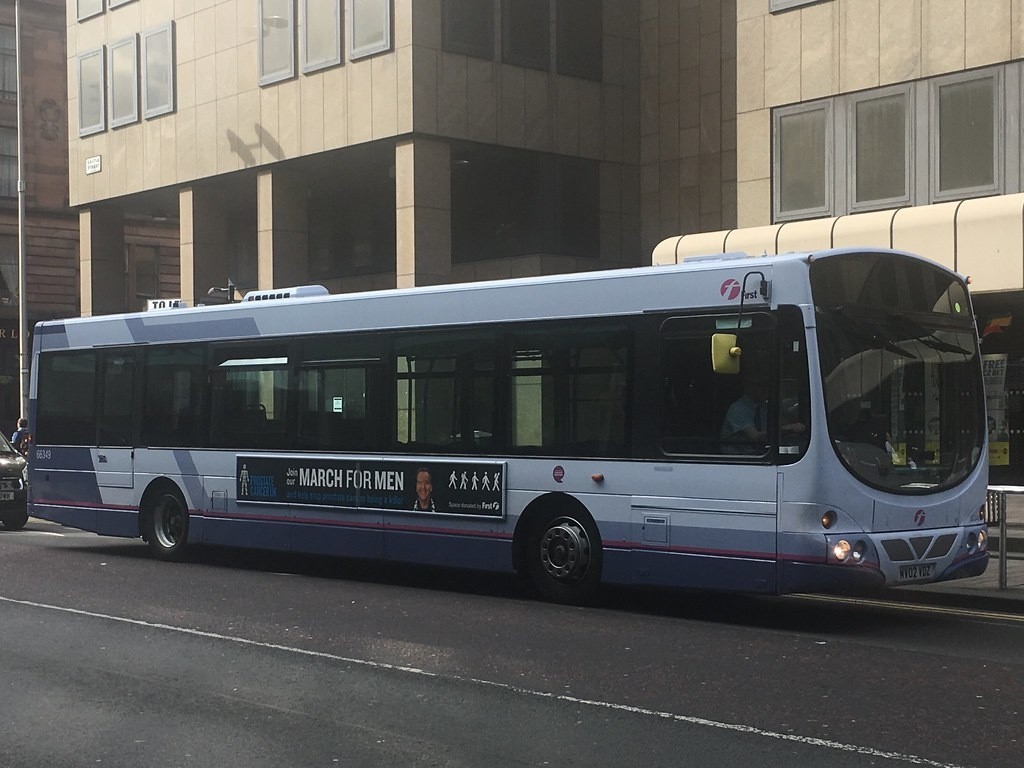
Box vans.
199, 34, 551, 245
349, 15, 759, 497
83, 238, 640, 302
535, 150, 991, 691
0, 430, 29, 528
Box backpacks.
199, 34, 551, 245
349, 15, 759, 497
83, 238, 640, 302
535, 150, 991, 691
18, 432, 29, 457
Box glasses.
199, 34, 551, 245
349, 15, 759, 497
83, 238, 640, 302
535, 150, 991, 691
748, 381, 767, 387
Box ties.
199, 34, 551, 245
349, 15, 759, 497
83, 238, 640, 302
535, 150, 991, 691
754, 406, 761, 432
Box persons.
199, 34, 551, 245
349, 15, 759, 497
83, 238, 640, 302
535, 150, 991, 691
719, 362, 807, 457
8, 417, 30, 457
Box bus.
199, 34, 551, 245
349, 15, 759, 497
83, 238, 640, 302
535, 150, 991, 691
27, 244, 990, 607
